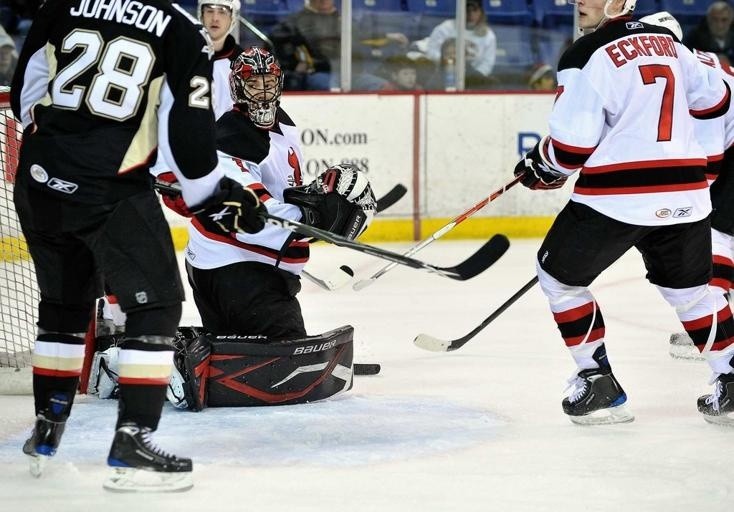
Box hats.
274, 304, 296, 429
524, 63, 552, 86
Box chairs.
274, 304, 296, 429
238, 1, 708, 91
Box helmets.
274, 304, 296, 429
567, 0, 637, 20
229, 45, 286, 130
199, 0, 241, 35
639, 11, 683, 42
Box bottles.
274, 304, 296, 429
444, 59, 456, 92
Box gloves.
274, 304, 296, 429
187, 175, 269, 235
514, 137, 569, 191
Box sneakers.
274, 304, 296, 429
672, 332, 697, 349
23, 391, 71, 458
107, 425, 192, 472
697, 355, 734, 416
562, 342, 626, 416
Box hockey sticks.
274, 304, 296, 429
375, 184, 407, 212
352, 172, 525, 293
301, 265, 355, 290
155, 179, 510, 281
413, 275, 539, 352
241, 19, 276, 53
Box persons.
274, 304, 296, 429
380, 1, 558, 94
513, 1, 733, 426
0, 0, 379, 490
